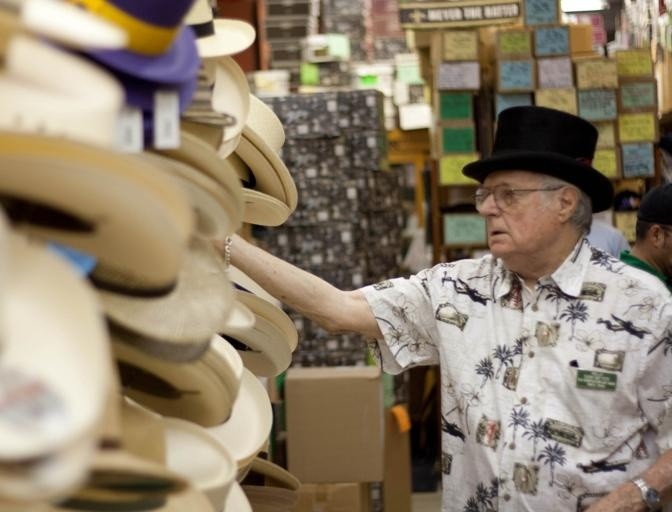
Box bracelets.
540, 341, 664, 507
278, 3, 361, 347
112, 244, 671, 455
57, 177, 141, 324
224, 235, 232, 269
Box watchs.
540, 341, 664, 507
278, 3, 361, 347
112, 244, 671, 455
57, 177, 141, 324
630, 474, 663, 512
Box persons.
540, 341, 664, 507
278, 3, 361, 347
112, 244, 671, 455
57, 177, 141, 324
619, 181, 672, 291
653, 104, 672, 183
587, 216, 631, 259
212, 103, 672, 512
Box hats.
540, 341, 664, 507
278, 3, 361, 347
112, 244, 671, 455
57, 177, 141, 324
0, 0, 298, 512
637, 183, 672, 225
462, 106, 614, 212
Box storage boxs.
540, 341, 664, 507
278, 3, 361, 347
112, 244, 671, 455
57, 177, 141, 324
282, 364, 386, 512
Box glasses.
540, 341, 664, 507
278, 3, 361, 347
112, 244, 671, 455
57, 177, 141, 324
475, 184, 565, 212
